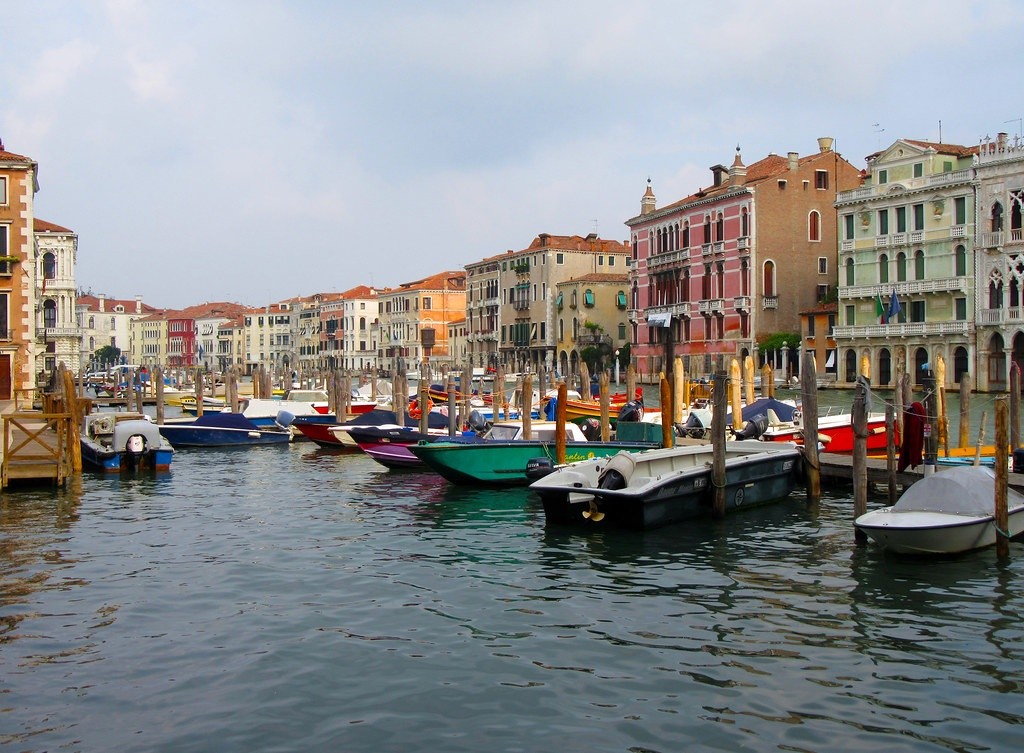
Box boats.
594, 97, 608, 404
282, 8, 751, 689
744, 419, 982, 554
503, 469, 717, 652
79, 404, 175, 474
853, 465, 1024, 556
529, 437, 827, 530
405, 420, 676, 487
84, 364, 1024, 468
148, 408, 297, 448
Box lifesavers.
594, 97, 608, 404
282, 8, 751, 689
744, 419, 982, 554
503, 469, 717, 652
408, 398, 432, 419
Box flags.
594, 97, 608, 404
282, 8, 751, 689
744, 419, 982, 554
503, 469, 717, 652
876, 288, 902, 324
198, 345, 204, 359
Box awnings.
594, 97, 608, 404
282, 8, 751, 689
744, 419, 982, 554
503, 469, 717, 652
556, 297, 563, 305
618, 295, 627, 306
585, 293, 594, 305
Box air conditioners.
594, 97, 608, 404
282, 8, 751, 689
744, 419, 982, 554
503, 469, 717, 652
643, 304, 691, 319
709, 301, 726, 316
626, 310, 638, 319
731, 297, 749, 309
585, 321, 599, 335
698, 301, 712, 317
